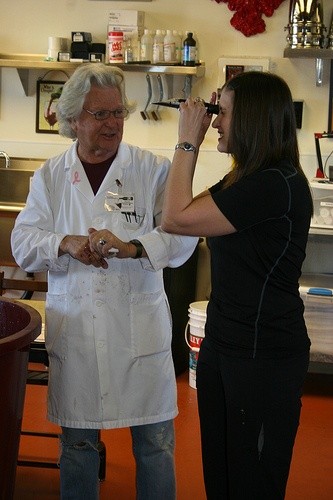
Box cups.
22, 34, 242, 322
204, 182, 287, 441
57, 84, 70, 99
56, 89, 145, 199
48, 37, 68, 62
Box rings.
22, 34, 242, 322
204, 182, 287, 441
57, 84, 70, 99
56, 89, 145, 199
99, 238, 105, 244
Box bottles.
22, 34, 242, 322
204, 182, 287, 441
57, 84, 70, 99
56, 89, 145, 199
140, 29, 197, 67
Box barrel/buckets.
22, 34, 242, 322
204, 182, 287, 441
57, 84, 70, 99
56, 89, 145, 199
184, 300, 210, 389
0, 295, 42, 500
284, 0, 327, 49
108, 32, 125, 63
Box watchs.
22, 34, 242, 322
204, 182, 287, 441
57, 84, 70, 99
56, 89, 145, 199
129, 239, 142, 258
175, 142, 196, 154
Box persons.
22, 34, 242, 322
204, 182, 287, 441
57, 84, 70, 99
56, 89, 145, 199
162, 71, 314, 500
10, 62, 199, 500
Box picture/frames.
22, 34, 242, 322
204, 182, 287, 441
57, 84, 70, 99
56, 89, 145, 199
219, 57, 270, 87
36, 79, 65, 133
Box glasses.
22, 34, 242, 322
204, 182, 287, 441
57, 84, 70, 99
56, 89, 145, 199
82, 106, 129, 120
